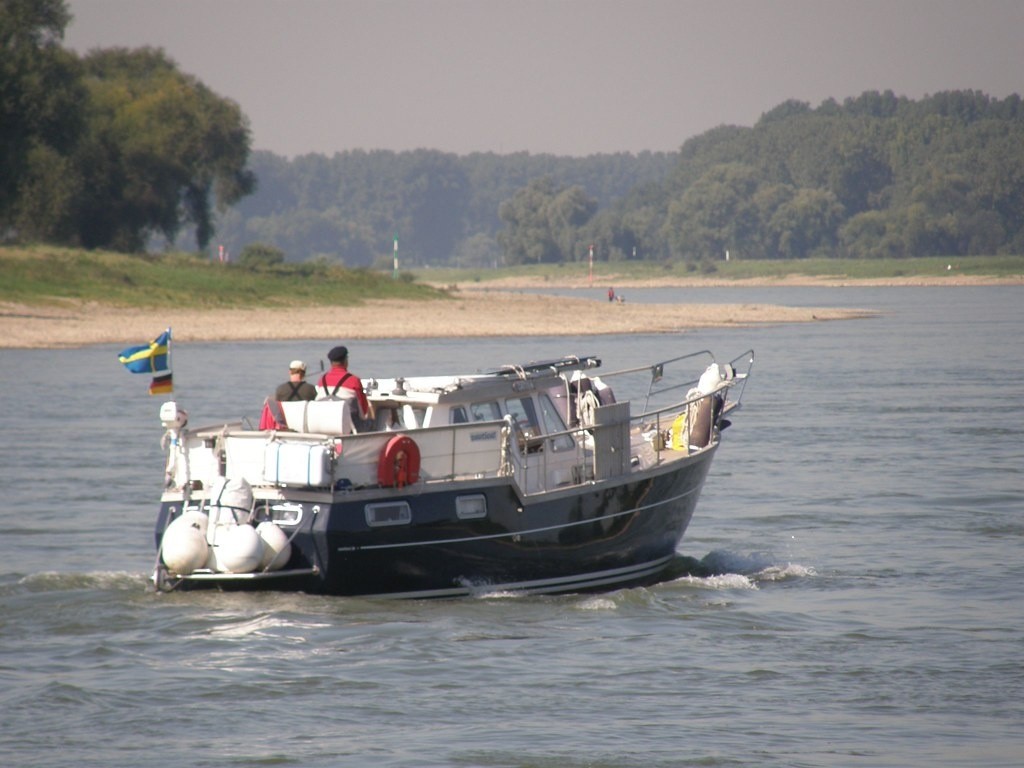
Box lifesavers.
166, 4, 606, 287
376, 433, 421, 486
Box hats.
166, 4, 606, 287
327, 346, 348, 360
289, 360, 306, 372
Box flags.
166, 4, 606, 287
149, 373, 173, 395
117, 331, 167, 374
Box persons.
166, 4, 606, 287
608, 287, 614, 302
275, 360, 318, 403
316, 346, 377, 433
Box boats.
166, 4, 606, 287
150, 349, 755, 598
